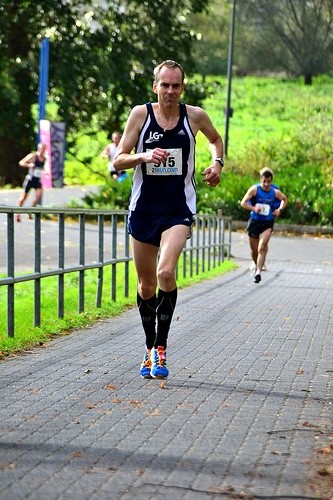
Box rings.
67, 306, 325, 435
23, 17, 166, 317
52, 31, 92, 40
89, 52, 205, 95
158, 155, 160, 159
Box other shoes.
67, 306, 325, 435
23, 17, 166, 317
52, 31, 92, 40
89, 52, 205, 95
17, 215, 20, 221
29, 214, 32, 219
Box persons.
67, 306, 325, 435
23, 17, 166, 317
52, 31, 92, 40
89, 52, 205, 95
241, 167, 287, 283
113, 60, 224, 380
15, 143, 46, 223
101, 131, 122, 177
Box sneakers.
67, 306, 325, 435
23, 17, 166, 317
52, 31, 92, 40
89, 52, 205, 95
150, 346, 169, 379
140, 349, 152, 378
253, 268, 261, 283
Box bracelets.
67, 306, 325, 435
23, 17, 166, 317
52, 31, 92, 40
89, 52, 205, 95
278, 208, 282, 212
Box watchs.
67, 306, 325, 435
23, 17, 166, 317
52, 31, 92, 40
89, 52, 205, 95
216, 157, 225, 166
